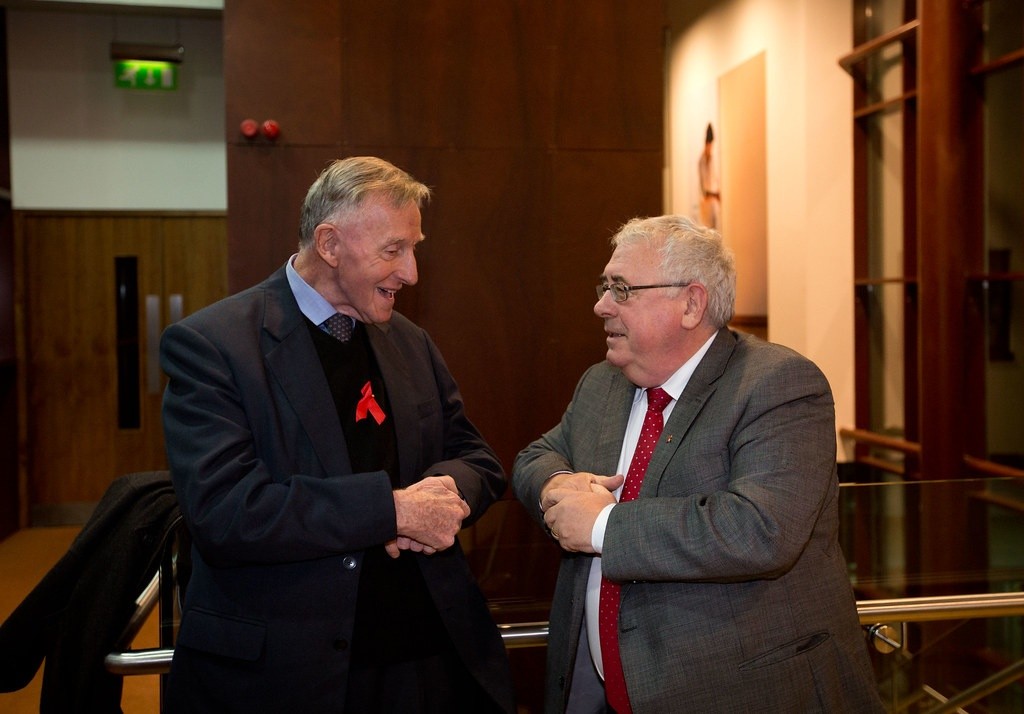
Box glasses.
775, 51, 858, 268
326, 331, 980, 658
595, 282, 693, 303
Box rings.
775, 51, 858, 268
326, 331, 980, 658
551, 528, 559, 536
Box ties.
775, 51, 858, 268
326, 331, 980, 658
598, 387, 673, 714
323, 312, 355, 343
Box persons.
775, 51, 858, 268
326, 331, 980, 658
512, 216, 887, 714
157, 156, 517, 714
